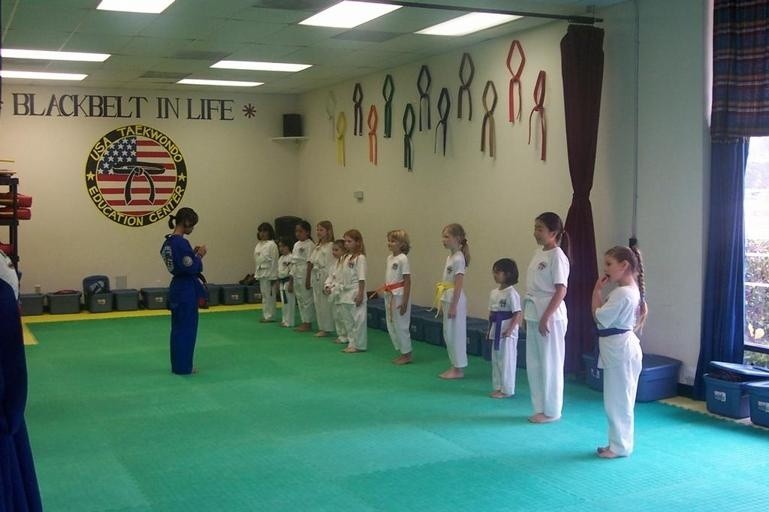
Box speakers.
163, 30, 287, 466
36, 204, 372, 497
283, 112, 302, 137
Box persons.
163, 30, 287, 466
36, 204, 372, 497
591, 245, 648, 458
324, 238, 347, 344
0, 277, 43, 512
160, 208, 208, 374
522, 212, 570, 424
276, 236, 296, 327
438, 223, 467, 380
291, 221, 315, 332
342, 229, 368, 353
488, 257, 523, 399
312, 220, 333, 337
253, 222, 279, 323
0, 249, 19, 301
383, 229, 413, 364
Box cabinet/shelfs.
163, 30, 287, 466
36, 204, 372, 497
0, 174, 23, 281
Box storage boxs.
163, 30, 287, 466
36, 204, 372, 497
579, 354, 682, 403
746, 381, 769, 427
703, 361, 769, 418
20, 280, 261, 316
368, 300, 534, 369
